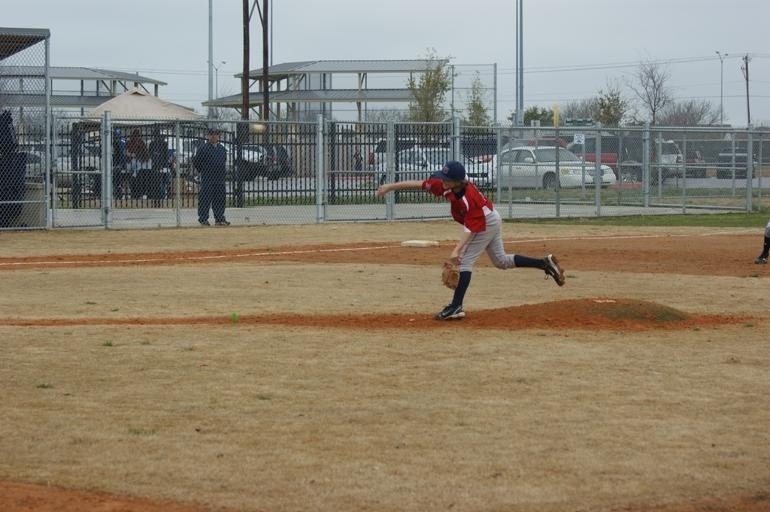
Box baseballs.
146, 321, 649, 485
252, 123, 265, 132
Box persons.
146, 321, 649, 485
754, 220, 770, 264
375, 161, 566, 321
113, 128, 170, 200
192, 128, 230, 226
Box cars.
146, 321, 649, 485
18, 130, 291, 187
715, 148, 757, 177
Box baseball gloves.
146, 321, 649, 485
440, 258, 459, 289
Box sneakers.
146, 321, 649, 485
753, 254, 769, 264
198, 218, 211, 226
215, 219, 231, 226
436, 305, 466, 320
544, 254, 565, 287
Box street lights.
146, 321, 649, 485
206, 59, 227, 119
715, 50, 730, 139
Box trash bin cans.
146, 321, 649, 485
16, 176, 45, 226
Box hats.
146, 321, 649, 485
431, 160, 465, 181
208, 128, 219, 134
